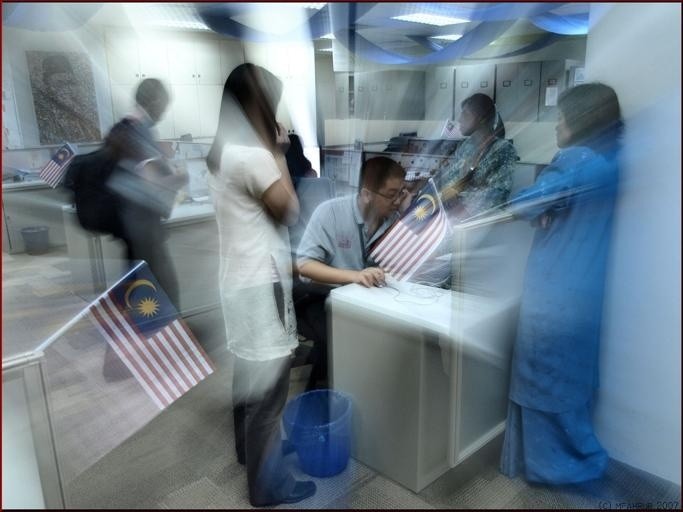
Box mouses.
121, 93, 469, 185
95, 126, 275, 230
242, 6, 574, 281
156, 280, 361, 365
373, 280, 386, 288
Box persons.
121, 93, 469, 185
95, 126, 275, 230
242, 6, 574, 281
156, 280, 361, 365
496, 84, 622, 484
296, 157, 413, 385
206, 62, 317, 507
103, 78, 190, 380
441, 95, 516, 221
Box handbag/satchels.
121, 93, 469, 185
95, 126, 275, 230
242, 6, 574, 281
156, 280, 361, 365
107, 157, 177, 217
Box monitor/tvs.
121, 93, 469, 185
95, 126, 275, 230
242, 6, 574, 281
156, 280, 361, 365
180, 156, 209, 199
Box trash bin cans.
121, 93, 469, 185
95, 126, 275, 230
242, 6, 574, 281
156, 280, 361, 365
282, 389, 352, 477
21, 226, 50, 256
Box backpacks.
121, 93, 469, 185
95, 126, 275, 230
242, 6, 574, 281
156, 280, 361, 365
65, 152, 112, 229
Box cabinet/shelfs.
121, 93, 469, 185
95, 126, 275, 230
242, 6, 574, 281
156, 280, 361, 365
333, 59, 580, 124
103, 24, 247, 143
2, 179, 76, 254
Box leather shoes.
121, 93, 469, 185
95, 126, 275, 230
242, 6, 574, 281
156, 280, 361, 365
282, 440, 294, 454
252, 482, 316, 505
103, 365, 130, 379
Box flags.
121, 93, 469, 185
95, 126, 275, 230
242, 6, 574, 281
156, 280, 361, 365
38, 142, 77, 189
82, 260, 216, 412
370, 177, 450, 286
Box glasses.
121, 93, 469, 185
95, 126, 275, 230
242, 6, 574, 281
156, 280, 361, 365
367, 187, 405, 201
